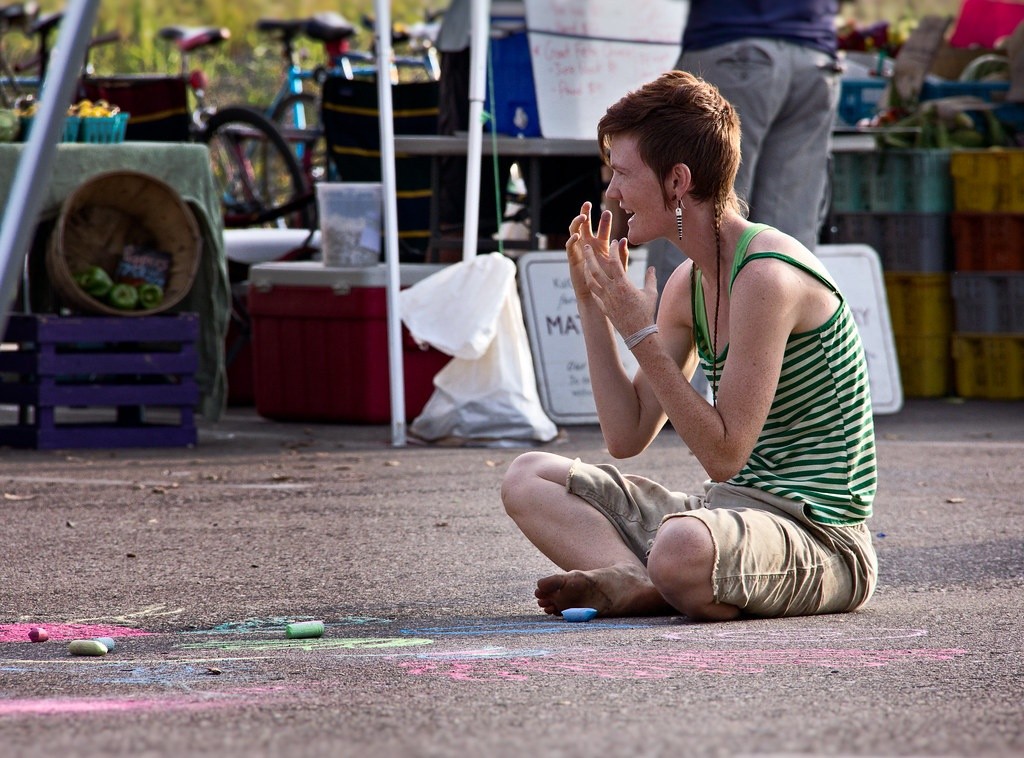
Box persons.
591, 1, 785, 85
499, 68, 878, 623
644, 0, 843, 430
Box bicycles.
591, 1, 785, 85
1, 1, 447, 229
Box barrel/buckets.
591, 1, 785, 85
45, 169, 205, 318
314, 179, 383, 270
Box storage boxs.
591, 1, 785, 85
818, 137, 1024, 399
245, 258, 450, 422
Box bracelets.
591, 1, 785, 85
623, 323, 659, 350
